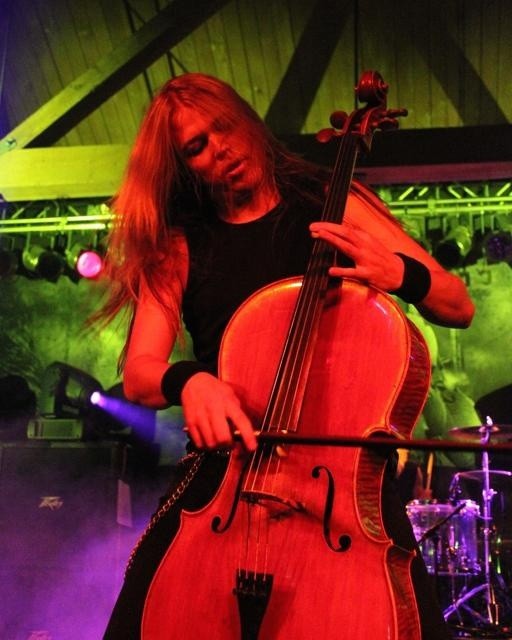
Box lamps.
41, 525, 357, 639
0, 229, 106, 282
40, 359, 108, 420
425, 213, 512, 268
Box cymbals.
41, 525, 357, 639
456, 471, 511, 486
450, 425, 512, 448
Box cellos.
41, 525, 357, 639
142, 69, 433, 639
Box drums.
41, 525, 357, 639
406, 500, 479, 580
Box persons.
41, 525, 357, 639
84, 67, 477, 640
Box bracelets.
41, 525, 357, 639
393, 255, 433, 306
157, 352, 210, 404
